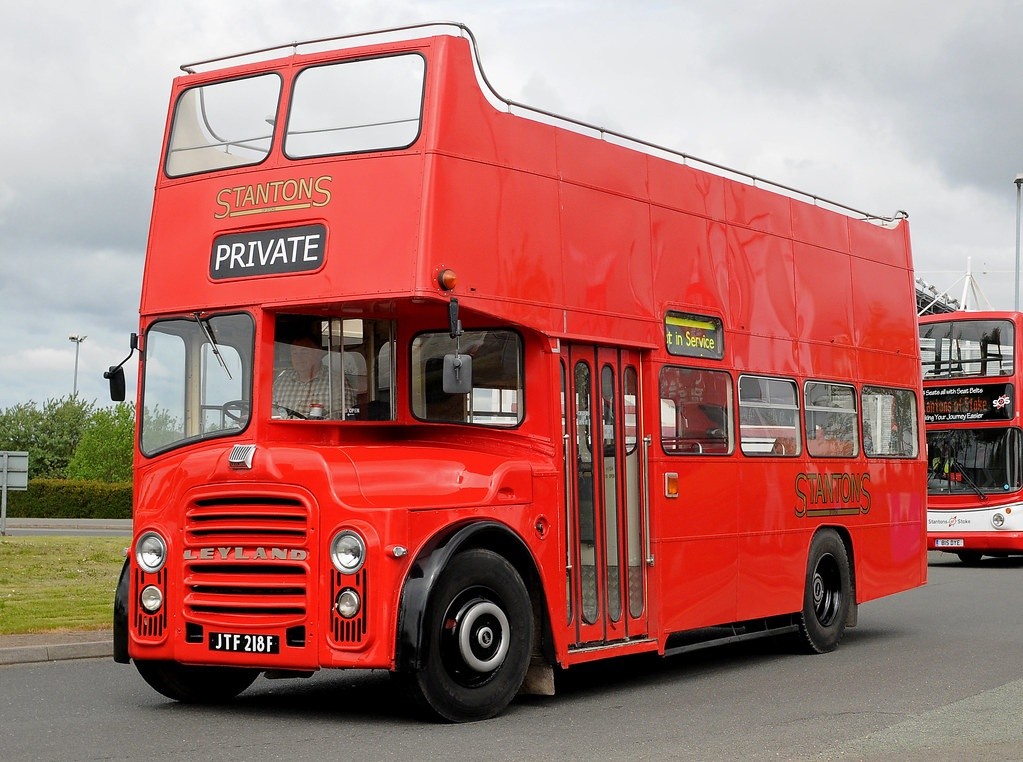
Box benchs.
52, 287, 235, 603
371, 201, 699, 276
808, 438, 852, 455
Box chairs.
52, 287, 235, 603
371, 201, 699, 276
770, 437, 797, 454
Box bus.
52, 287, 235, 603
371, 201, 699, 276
916, 312, 1023, 564
112, 21, 928, 725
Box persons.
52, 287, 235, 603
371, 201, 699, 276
271, 336, 356, 423
932, 443, 954, 473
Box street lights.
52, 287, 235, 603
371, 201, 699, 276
69, 334, 87, 399
